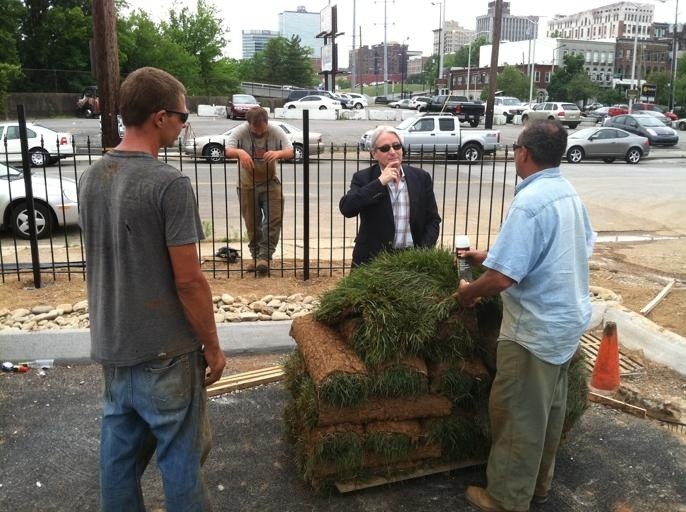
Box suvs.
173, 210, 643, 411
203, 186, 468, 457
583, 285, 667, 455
632, 103, 678, 121
485, 97, 530, 123
347, 93, 368, 110
287, 90, 354, 109
521, 102, 582, 129
408, 96, 430, 110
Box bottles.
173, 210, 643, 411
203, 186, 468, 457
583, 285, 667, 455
456, 236, 471, 280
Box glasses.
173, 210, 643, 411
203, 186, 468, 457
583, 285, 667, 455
376, 141, 403, 152
512, 141, 524, 152
161, 108, 190, 124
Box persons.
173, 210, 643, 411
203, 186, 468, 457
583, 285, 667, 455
455, 114, 595, 512
223, 106, 297, 272
74, 66, 226, 511
336, 124, 443, 277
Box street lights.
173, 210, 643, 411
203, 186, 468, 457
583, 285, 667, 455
524, 17, 544, 105
432, 0, 445, 78
402, 34, 410, 100
373, 0, 396, 100
619, 0, 666, 119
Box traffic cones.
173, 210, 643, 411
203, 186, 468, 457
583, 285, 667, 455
589, 321, 621, 398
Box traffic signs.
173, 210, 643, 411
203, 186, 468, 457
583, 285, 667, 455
641, 83, 657, 104
626, 88, 639, 100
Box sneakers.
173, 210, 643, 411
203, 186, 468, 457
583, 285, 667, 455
465, 484, 502, 511
532, 492, 549, 506
247, 257, 256, 272
256, 258, 268, 272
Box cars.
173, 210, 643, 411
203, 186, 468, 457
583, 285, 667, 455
225, 93, 260, 120
604, 114, 679, 147
632, 110, 672, 129
72, 86, 102, 119
672, 119, 686, 131
184, 121, 324, 164
0, 162, 79, 240
561, 127, 651, 164
608, 108, 628, 117
583, 103, 603, 110
388, 99, 411, 109
335, 94, 353, 108
1, 122, 76, 167
283, 95, 342, 110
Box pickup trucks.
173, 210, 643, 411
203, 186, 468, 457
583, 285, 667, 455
420, 95, 485, 128
361, 113, 503, 165
